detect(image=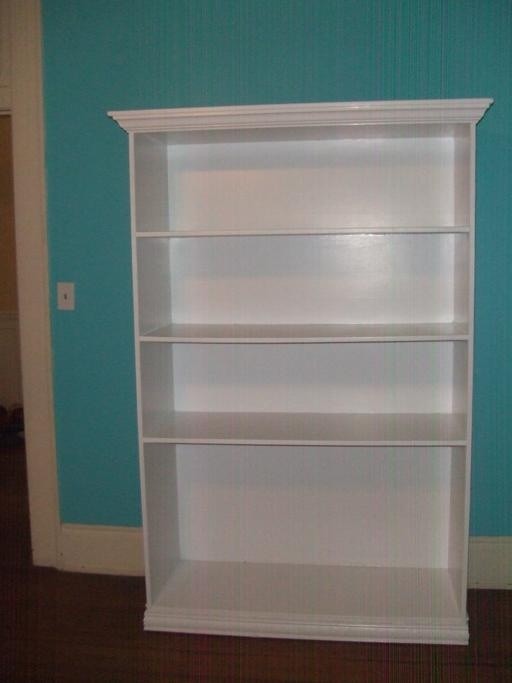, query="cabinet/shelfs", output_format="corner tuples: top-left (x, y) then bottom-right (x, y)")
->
(106, 96), (496, 646)
